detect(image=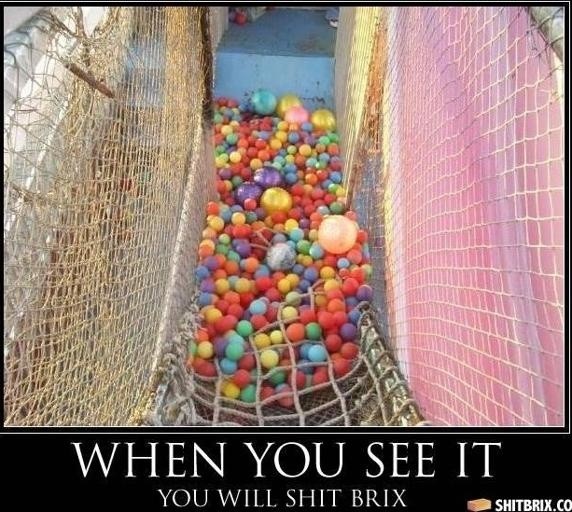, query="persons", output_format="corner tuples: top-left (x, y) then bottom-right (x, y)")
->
(188, 91), (374, 408)
(186, 91), (373, 408)
